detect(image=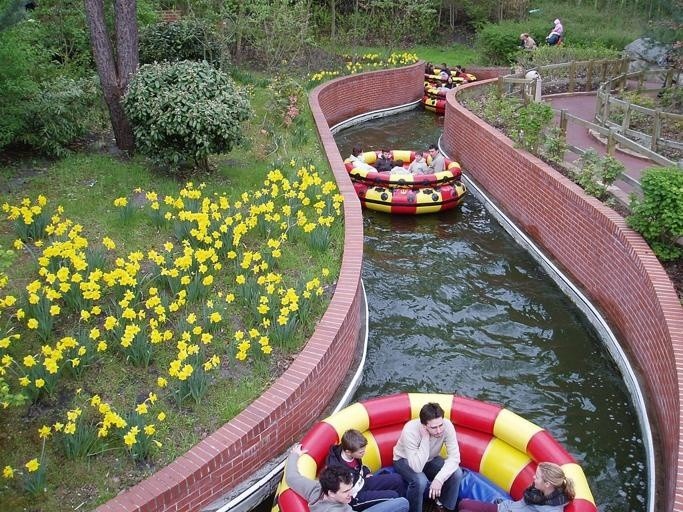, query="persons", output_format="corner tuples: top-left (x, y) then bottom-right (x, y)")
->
(390, 159), (410, 174)
(391, 401), (464, 512)
(325, 429), (405, 509)
(457, 462), (575, 512)
(427, 144), (447, 173)
(376, 146), (394, 171)
(520, 33), (537, 49)
(545, 18), (564, 45)
(349, 145), (378, 171)
(426, 61), (471, 90)
(283, 443), (410, 512)
(407, 152), (427, 172)
